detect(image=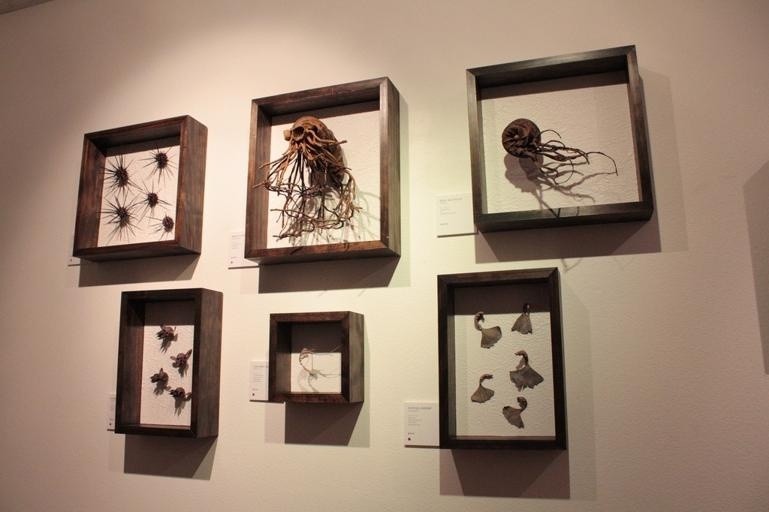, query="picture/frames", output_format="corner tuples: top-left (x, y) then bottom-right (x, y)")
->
(71, 44), (654, 450)
